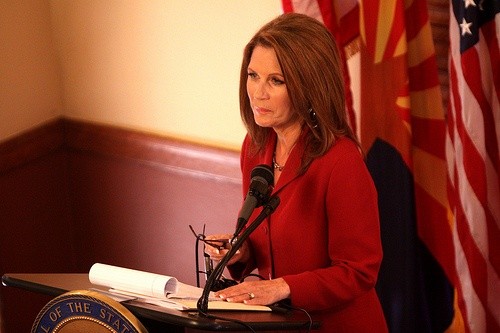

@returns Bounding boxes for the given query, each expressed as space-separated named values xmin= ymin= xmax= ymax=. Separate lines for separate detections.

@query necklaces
xmin=273 ymin=153 xmax=285 ymax=172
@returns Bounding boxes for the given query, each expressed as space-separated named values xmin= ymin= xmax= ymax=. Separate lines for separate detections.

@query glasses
xmin=189 ymin=224 xmax=237 ymax=250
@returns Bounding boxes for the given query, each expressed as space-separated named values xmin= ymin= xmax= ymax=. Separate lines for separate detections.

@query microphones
xmin=234 ymin=164 xmax=274 ymax=237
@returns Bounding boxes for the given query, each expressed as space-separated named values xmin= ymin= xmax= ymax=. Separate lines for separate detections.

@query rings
xmin=248 ymin=292 xmax=256 ymax=300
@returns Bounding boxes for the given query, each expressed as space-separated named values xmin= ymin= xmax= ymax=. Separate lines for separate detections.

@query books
xmin=89 ymin=262 xmax=272 ymax=312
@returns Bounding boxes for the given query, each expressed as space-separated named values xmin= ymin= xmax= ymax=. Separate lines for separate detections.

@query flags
xmin=282 ymin=0 xmax=500 ymax=333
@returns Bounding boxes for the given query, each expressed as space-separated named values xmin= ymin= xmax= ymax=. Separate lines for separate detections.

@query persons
xmin=203 ymin=12 xmax=384 ymax=333
